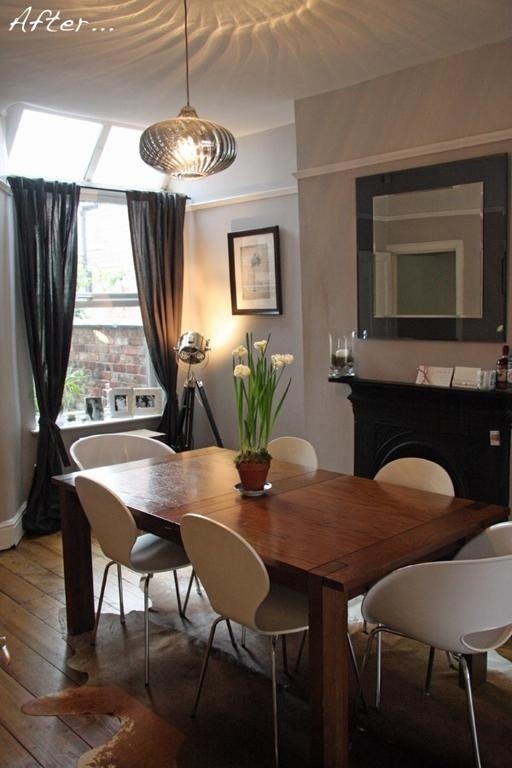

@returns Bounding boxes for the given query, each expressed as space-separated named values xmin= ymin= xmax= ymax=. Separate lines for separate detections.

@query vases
xmin=233 ymin=448 xmax=273 ymax=497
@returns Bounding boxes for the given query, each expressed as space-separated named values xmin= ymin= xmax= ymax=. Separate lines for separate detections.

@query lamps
xmin=138 ymin=2 xmax=237 ymax=181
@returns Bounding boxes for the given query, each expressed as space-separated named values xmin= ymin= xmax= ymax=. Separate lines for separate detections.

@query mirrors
xmin=370 ymin=182 xmax=486 ymax=321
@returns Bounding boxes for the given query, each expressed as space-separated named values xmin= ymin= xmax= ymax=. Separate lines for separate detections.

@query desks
xmin=54 ymin=444 xmax=502 ymax=768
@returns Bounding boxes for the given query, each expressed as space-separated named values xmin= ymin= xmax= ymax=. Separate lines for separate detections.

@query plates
xmin=234 ymin=482 xmax=272 ymax=497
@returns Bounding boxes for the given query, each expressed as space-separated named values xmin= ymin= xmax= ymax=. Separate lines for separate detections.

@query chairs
xmin=179 ymin=512 xmax=358 ymax=768
xmin=454 ymin=520 xmax=512 ymax=560
xmin=74 ymin=475 xmax=238 ymax=687
xmin=373 ymin=456 xmax=454 ymax=499
xmin=69 ymin=434 xmax=200 ymax=613
xmin=358 ymin=555 xmax=511 ymax=768
xmin=265 ymin=435 xmax=317 ymax=469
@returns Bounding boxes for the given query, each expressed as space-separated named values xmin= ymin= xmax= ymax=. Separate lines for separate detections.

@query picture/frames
xmin=226 ymin=225 xmax=284 ymax=317
xmin=83 ymin=396 xmax=104 ymax=422
xmin=131 ymin=388 xmax=162 ymax=415
xmin=107 ymin=387 xmax=133 ymax=417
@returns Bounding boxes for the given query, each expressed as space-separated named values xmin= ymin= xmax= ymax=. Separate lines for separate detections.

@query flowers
xmin=230 ymin=332 xmax=294 ymax=456
